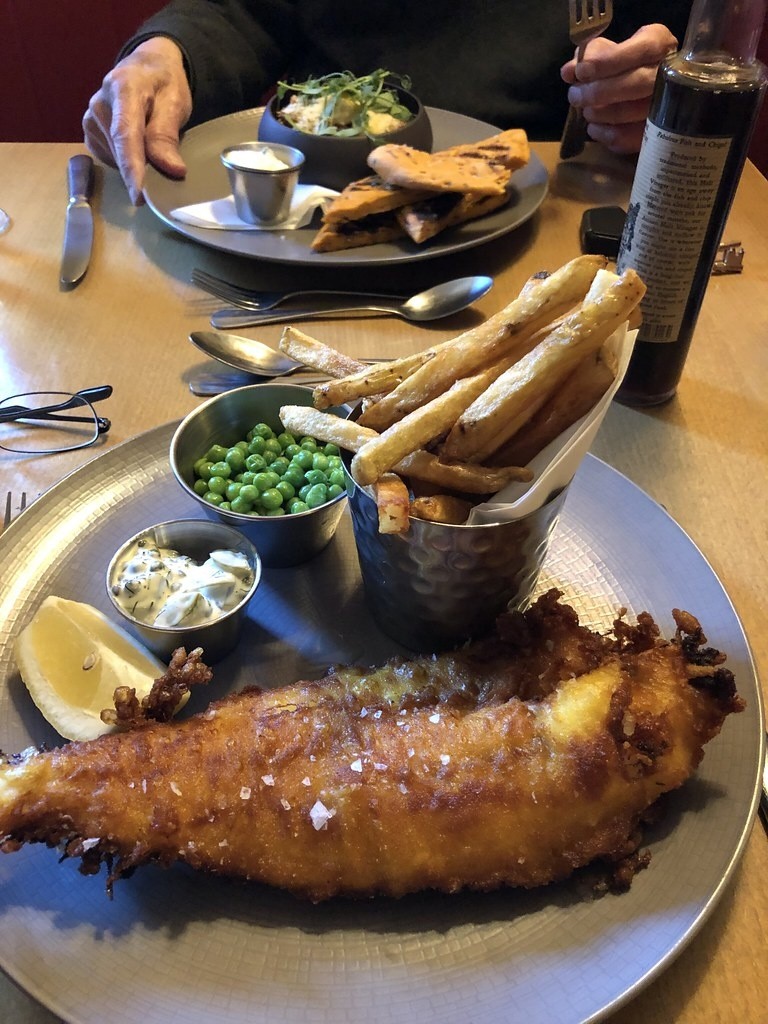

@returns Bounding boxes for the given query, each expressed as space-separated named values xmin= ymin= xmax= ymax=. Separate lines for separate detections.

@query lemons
xmin=13 ymin=594 xmax=194 ymax=746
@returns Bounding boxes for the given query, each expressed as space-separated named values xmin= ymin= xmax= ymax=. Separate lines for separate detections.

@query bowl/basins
xmin=258 ymin=79 xmax=433 ymax=192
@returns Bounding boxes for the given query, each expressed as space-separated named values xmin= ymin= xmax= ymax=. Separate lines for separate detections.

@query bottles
xmin=602 ymin=1 xmax=768 ymax=408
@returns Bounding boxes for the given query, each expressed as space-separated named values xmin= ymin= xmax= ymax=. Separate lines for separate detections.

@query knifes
xmin=59 ymin=154 xmax=94 ymax=284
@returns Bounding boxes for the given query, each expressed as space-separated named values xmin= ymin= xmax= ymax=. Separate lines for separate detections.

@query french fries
xmin=279 ymin=254 xmax=645 ymax=538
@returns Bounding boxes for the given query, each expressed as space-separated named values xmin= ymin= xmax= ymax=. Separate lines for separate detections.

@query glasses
xmin=0 ymin=383 xmax=112 ymax=454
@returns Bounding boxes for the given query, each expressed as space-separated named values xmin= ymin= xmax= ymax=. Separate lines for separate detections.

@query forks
xmin=189 ymin=267 xmax=414 ymax=312
xmin=559 ymin=0 xmax=613 ymax=158
xmin=4 ymin=491 xmax=41 ymax=531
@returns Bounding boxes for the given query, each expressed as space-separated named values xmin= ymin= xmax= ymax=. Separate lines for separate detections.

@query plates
xmin=143 ymin=104 xmax=550 ymax=266
xmin=1 ymin=404 xmax=764 ymax=1023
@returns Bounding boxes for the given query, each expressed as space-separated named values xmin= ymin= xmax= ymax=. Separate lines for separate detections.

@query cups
xmin=220 ymin=140 xmax=305 ymax=226
xmin=106 ymin=517 xmax=263 ymax=667
xmin=339 ymin=402 xmax=576 ymax=652
xmin=170 ymin=385 xmax=358 ymax=565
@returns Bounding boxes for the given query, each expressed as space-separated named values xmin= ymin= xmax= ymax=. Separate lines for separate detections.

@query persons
xmin=81 ymin=0 xmax=694 ymax=207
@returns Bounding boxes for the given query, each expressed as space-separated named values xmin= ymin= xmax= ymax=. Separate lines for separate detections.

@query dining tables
xmin=2 ymin=127 xmax=768 ymax=1024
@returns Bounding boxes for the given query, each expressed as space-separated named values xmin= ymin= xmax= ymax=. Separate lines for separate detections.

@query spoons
xmin=189 ymin=331 xmax=398 ymax=376
xmin=210 ymin=275 xmax=493 ymax=329
